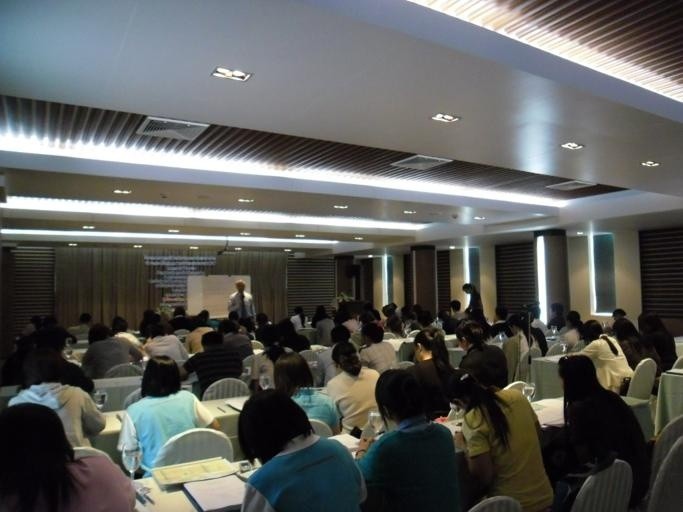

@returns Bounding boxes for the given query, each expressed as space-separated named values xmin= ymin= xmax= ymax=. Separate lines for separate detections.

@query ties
xmin=241 ymin=295 xmax=246 ymax=319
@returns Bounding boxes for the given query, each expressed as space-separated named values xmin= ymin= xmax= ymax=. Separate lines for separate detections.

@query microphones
xmin=239 ymin=287 xmax=244 ymax=298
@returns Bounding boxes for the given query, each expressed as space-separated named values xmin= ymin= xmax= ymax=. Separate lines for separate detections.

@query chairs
xmin=0 ymin=320 xmax=683 ymax=512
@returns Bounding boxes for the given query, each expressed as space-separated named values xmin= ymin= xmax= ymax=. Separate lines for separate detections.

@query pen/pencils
xmin=144 ymin=495 xmax=155 ymax=506
xmin=217 ymin=407 xmax=226 ymax=413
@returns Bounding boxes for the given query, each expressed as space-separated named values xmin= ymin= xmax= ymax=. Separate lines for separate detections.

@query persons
xmin=1 ymin=277 xmax=682 ymax=512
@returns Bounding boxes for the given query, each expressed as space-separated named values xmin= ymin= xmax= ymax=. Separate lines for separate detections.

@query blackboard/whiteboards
xmin=186 ymin=275 xmax=251 ymax=319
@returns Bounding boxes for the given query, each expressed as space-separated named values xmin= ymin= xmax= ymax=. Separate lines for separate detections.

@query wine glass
xmin=92 ymin=390 xmax=107 ymax=412
xmin=64 ymin=347 xmax=73 ymax=361
xmin=122 ymin=440 xmax=143 ymax=492
xmin=367 ymin=409 xmax=384 ymax=437
xmin=258 ymin=375 xmax=270 ymax=390
xmin=551 ymin=326 xmax=557 ymax=338
xmin=498 ymin=331 xmax=504 ymax=343
xmin=523 ymin=381 xmax=536 ymax=403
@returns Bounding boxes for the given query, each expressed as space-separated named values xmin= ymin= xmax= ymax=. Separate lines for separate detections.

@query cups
xmin=560 ymin=342 xmax=567 ymax=353
